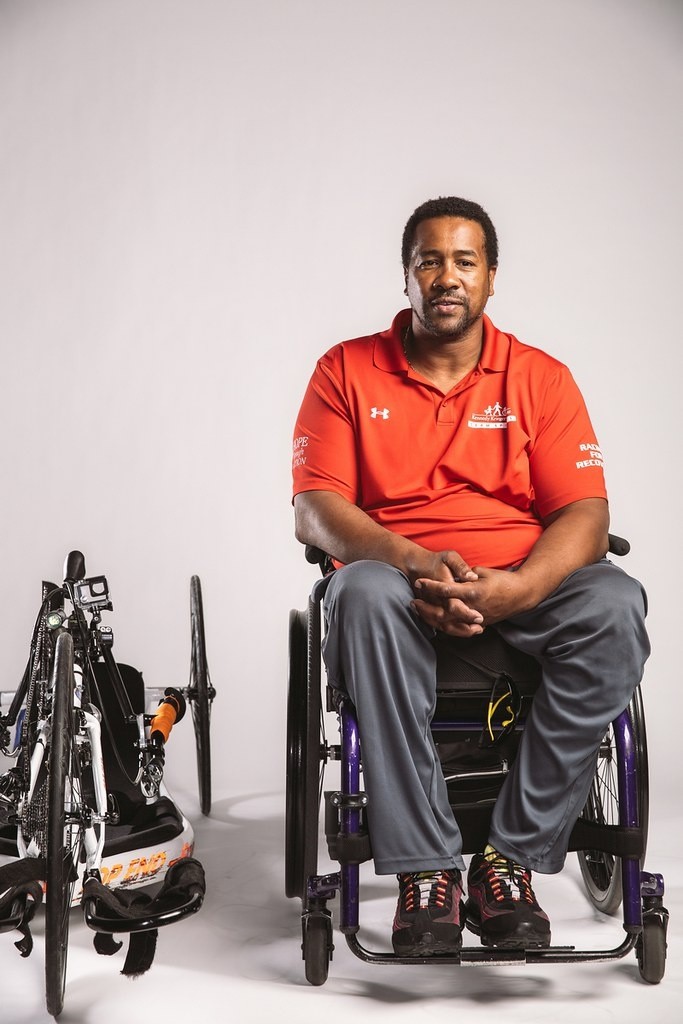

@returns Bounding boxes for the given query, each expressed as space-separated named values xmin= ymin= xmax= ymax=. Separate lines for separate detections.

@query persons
xmin=288 ymin=196 xmax=652 ymax=959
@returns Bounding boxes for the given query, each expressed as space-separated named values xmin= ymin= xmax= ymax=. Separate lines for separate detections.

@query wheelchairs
xmin=0 ymin=550 xmax=217 ymax=1015
xmin=284 ymin=536 xmax=670 ymax=986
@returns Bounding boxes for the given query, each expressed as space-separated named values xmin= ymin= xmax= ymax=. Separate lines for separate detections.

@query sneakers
xmin=392 ymin=868 xmax=462 ymax=953
xmin=462 ymin=849 xmax=552 ymax=949
